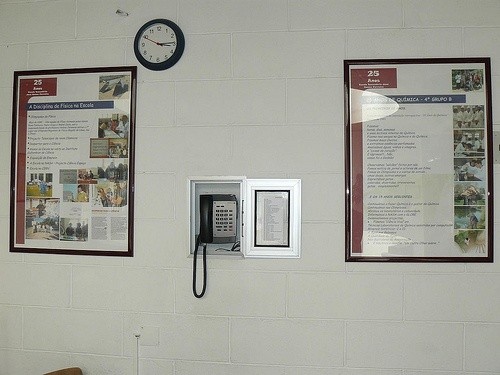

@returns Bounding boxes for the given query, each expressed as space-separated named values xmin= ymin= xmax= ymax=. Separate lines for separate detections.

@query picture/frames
xmin=343 ymin=58 xmax=496 ymax=264
xmin=8 ymin=66 xmax=139 ymax=258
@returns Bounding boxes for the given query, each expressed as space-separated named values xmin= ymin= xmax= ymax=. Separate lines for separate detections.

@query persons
xmin=26 ymin=112 xmax=129 ymax=242
xmin=452 ymin=69 xmax=488 ymax=252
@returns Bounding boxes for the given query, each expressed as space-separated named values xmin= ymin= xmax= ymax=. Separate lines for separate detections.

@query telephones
xmin=199 ymin=194 xmax=238 ymax=244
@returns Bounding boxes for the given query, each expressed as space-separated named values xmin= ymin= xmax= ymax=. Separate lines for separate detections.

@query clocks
xmin=131 ymin=18 xmax=187 ymax=73
